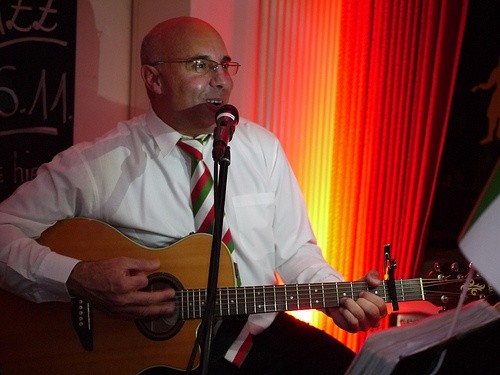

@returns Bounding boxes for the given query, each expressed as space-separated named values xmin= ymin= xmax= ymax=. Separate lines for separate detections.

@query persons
xmin=0 ymin=16 xmax=386 ymax=375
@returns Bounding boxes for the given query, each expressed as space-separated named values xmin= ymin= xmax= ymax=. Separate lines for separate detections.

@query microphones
xmin=211 ymin=104 xmax=239 ymax=162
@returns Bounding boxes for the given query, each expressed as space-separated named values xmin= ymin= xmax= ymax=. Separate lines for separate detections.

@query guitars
xmin=0 ymin=215 xmax=488 ymax=375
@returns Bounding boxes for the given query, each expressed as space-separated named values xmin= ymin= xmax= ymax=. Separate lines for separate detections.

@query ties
xmin=176 ymin=135 xmax=242 ymax=287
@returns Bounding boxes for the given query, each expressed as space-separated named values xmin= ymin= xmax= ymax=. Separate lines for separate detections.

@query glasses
xmin=153 ymin=58 xmax=241 ymax=77
